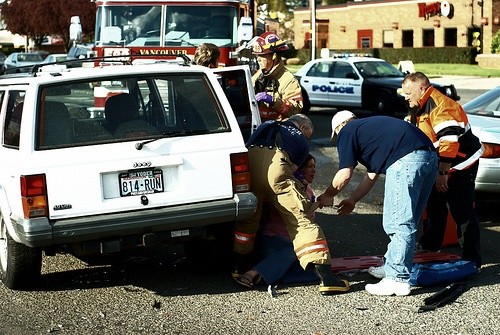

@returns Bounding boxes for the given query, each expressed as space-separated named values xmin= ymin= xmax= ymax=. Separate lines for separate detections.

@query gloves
xmin=255 ymin=92 xmax=272 ymax=103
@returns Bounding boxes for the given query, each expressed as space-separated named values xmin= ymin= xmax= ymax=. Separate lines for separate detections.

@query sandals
xmin=233 ymin=274 xmax=254 ymax=288
xmin=255 ymin=276 xmax=262 ymax=285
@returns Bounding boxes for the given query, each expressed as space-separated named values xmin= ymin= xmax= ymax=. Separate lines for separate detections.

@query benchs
xmin=43 ymin=101 xmax=161 ymax=145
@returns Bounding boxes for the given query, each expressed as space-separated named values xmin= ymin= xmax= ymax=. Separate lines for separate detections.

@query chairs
xmin=101 ymin=93 xmax=139 ymax=134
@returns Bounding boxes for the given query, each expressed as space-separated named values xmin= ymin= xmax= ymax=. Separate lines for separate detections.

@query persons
xmin=232 ymin=114 xmax=350 ymax=295
xmin=400 ymin=71 xmax=485 ymax=270
xmin=251 ymin=32 xmax=304 ymax=124
xmin=193 ymin=42 xmax=230 ymax=99
xmin=317 ymin=110 xmax=440 ymax=297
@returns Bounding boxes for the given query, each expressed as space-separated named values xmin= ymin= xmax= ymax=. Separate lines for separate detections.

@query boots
xmin=313 ymin=264 xmax=350 ymax=295
xmin=231 ymin=251 xmax=252 ymax=277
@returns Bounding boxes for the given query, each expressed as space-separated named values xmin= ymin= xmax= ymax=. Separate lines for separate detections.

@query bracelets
xmin=439 ymin=170 xmax=448 ymax=175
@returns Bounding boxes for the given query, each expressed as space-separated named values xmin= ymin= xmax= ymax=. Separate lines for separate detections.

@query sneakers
xmin=368 ymin=265 xmax=386 ymax=278
xmin=365 ymin=278 xmax=411 ymax=296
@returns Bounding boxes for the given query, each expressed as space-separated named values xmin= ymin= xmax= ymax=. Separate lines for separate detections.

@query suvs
xmin=0 ymin=48 xmax=266 ymax=290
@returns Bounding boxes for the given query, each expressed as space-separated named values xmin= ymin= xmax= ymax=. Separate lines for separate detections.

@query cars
xmin=0 ymin=42 xmax=94 ymax=77
xmin=293 ymin=47 xmax=462 ymax=119
xmin=461 ymin=86 xmax=500 ymax=193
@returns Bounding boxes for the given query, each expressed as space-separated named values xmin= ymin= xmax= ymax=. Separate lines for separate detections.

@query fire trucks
xmin=85 ymin=0 xmax=276 ymax=118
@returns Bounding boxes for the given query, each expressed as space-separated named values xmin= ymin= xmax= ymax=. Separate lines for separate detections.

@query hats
xmin=330 ymin=110 xmax=356 ymax=142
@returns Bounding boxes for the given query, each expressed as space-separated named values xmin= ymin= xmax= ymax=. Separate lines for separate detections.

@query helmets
xmin=252 ymin=32 xmax=288 ymax=55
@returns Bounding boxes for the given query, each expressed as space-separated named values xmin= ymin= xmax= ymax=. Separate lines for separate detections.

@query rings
xmin=442 ymin=185 xmax=444 ymax=188
